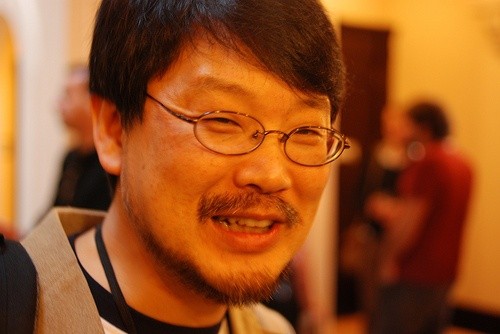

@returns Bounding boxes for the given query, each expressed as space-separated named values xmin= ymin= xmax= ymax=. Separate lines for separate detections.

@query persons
xmin=0 ymin=0 xmax=347 ymax=334
xmin=364 ymin=100 xmax=475 ymax=334
xmin=30 ymin=70 xmax=116 ymax=232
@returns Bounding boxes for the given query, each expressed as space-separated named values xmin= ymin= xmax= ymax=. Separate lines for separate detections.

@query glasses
xmin=144 ymin=91 xmax=352 ymax=168
